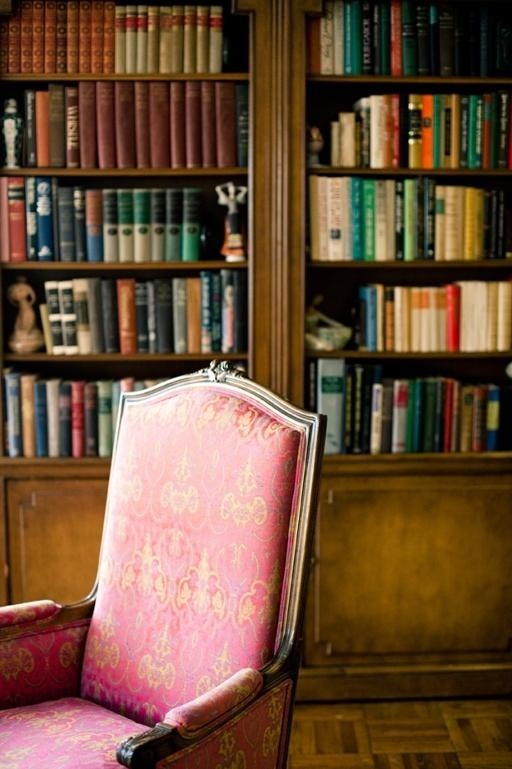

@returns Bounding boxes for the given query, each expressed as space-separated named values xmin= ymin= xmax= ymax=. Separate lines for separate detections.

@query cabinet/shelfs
xmin=0 ymin=1 xmax=512 ymax=457
xmin=0 ymin=458 xmax=512 ymax=698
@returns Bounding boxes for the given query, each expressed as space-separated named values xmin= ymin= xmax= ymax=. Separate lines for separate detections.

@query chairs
xmin=1 ymin=367 xmax=324 ymax=768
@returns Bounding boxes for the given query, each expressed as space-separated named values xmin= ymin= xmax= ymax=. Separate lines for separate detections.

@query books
xmin=352 ymin=279 xmax=509 ymax=353
xmin=0 ymin=174 xmax=210 ymax=263
xmin=3 ymin=78 xmax=247 ymax=172
xmin=325 ymin=80 xmax=511 ymax=166
xmin=38 ymin=272 xmax=249 ymax=362
xmin=1 ymin=0 xmax=250 ymax=74
xmin=309 ymin=2 xmax=510 ymax=75
xmin=305 ymin=175 xmax=509 ymax=263
xmin=2 ymin=373 xmax=188 ymax=458
xmin=304 ymin=358 xmax=512 ymax=455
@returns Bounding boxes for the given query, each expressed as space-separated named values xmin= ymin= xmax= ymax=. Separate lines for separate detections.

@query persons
xmin=305 ymin=292 xmax=351 ymax=352
xmin=214 ymin=180 xmax=246 ymax=262
xmin=3 ymin=274 xmax=42 ymax=356
